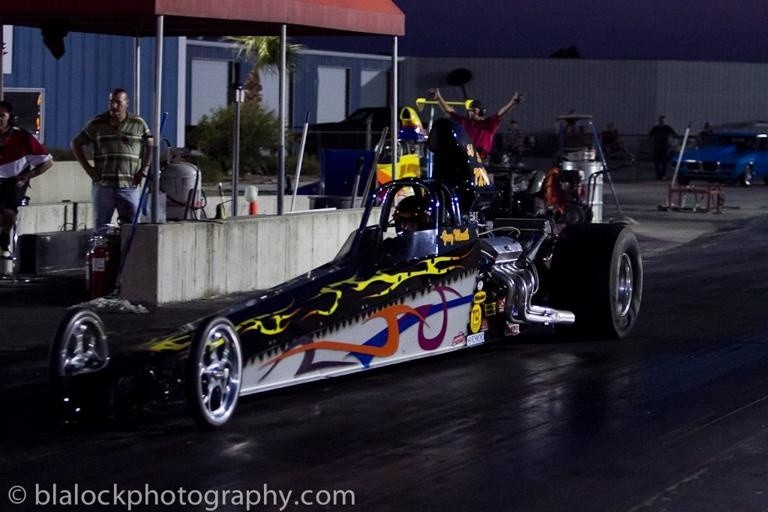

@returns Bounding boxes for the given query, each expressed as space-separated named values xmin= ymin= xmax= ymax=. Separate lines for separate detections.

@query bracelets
xmin=138 ymin=170 xmax=149 ymax=177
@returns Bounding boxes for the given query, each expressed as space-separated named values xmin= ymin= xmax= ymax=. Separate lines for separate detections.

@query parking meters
xmin=229 ymin=82 xmax=247 ymax=220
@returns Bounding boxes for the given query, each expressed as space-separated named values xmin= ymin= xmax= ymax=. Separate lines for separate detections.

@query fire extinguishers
xmin=90 ymin=234 xmax=106 ymax=298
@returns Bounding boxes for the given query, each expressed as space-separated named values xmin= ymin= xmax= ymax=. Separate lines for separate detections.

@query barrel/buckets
xmin=561 ymin=160 xmax=604 ymax=223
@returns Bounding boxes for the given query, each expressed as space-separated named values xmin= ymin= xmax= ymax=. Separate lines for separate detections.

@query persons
xmin=0 ymin=97 xmax=55 ymax=262
xmin=697 ymin=121 xmax=716 ymax=145
xmin=646 ymin=115 xmax=682 ymax=168
xmin=390 ymin=193 xmax=438 ymax=246
xmin=68 ymin=87 xmax=156 ymax=228
xmin=425 ymin=84 xmax=524 ymax=168
xmin=597 ymin=121 xmax=625 ymax=162
xmin=500 ymin=119 xmax=527 ymax=157
xmin=562 ymin=109 xmax=578 ymax=138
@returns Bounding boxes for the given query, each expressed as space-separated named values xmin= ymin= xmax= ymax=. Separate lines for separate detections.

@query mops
xmin=63 ymin=111 xmax=167 ymax=314
xmin=590 ymin=120 xmax=641 ymax=225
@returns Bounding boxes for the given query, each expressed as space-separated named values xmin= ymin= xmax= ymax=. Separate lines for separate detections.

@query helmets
xmin=392 ymin=195 xmax=432 ymax=243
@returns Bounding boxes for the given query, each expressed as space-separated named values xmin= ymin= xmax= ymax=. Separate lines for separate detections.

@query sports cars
xmin=37 ymin=112 xmax=651 ymax=432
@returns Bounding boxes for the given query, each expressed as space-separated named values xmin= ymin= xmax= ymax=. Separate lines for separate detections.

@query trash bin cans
xmin=328 ymin=196 xmax=363 ymax=208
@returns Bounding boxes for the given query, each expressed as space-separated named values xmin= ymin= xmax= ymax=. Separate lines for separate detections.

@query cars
xmin=669 ymin=119 xmax=768 ymax=188
